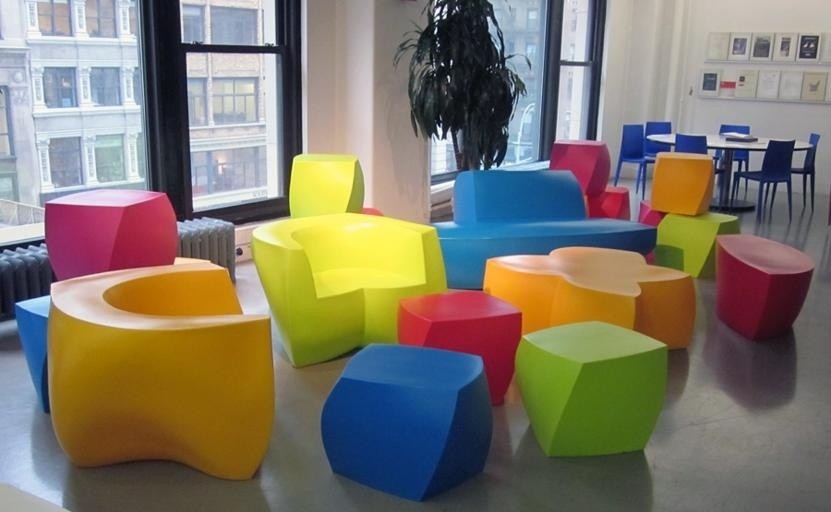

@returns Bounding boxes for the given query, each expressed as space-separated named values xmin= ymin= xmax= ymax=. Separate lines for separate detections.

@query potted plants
xmin=392 ymin=1 xmax=531 ymax=212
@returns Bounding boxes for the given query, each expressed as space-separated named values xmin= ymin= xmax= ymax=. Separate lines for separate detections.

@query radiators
xmin=0 ymin=216 xmax=241 ymax=322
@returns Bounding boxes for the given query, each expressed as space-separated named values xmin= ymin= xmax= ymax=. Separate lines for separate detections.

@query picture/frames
xmin=705 ymin=30 xmax=821 ymax=65
xmin=697 ymin=67 xmax=831 ymax=104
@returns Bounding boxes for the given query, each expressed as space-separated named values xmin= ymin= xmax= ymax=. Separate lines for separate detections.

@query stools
xmin=713 ymin=232 xmax=813 ymax=341
xmin=325 ymin=344 xmax=496 ymax=501
xmin=654 ymin=212 xmax=738 ymax=279
xmin=288 ymin=153 xmax=365 ymax=217
xmin=399 ymin=289 xmax=523 ymax=406
xmin=638 ymin=198 xmax=661 ymax=264
xmin=590 ymin=187 xmax=630 ymax=221
xmin=481 ymin=246 xmax=697 ymax=352
xmin=15 ymin=296 xmax=53 ymax=413
xmin=551 ymin=140 xmax=611 ymax=193
xmin=516 ymin=321 xmax=669 ymax=458
xmin=43 ymin=187 xmax=178 ymax=281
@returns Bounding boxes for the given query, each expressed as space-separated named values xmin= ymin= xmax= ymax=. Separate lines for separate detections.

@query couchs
xmin=429 ymin=169 xmax=656 ymax=291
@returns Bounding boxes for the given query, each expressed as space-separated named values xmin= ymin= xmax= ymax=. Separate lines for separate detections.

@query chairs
xmin=47 ymin=258 xmax=275 ymax=481
xmin=615 ymin=122 xmax=820 ymax=223
xmin=250 ymin=211 xmax=448 ymax=370
